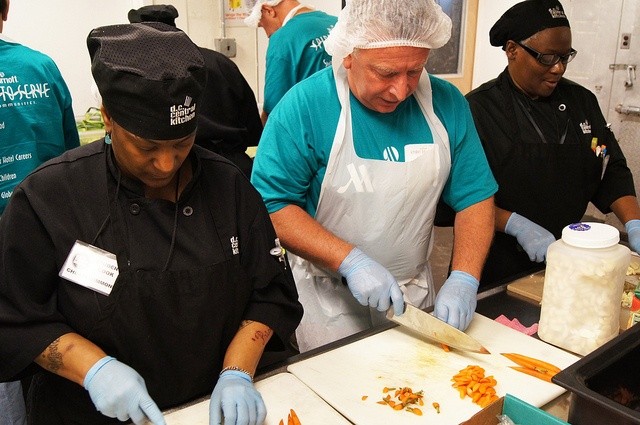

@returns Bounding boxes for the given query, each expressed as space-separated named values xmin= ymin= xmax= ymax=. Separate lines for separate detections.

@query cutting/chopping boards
xmin=507 ymin=254 xmax=639 ymax=334
xmin=287 ymin=310 xmax=581 ymax=425
xmin=145 ymin=372 xmax=353 ymax=425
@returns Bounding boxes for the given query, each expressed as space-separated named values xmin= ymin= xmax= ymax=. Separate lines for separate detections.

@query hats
xmin=244 ymin=0 xmax=283 ymax=29
xmin=489 ymin=0 xmax=570 ymax=51
xmin=128 ymin=5 xmax=178 ymax=28
xmin=323 ymin=0 xmax=452 ymax=58
xmin=87 ymin=22 xmax=206 ymax=140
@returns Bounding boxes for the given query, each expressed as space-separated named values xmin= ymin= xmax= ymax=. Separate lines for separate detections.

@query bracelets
xmin=219 ymin=366 xmax=255 ymax=383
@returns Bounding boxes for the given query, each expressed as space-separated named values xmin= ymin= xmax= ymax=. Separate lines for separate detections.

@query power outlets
xmin=620 ymin=32 xmax=631 ymax=50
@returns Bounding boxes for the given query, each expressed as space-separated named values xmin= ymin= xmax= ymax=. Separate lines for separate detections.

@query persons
xmin=1 ymin=0 xmax=80 ymax=214
xmin=0 ymin=382 xmax=28 ymax=425
xmin=449 ymin=0 xmax=640 ymax=293
xmin=246 ymin=0 xmax=339 ymax=127
xmin=1 ymin=21 xmax=305 ymax=425
xmin=128 ymin=4 xmax=262 ymax=183
xmin=250 ymin=1 xmax=500 ymax=355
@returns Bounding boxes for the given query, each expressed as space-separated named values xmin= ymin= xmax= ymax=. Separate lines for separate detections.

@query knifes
xmin=341 ymin=276 xmax=491 ymax=354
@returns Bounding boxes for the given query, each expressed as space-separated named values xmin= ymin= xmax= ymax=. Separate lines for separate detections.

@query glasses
xmin=516 ymin=42 xmax=577 ymax=66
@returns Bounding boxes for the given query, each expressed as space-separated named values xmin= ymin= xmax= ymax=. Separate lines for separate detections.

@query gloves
xmin=338 ymin=247 xmax=404 ymax=316
xmin=434 ymin=270 xmax=479 ymax=332
xmin=209 ymin=370 xmax=267 ymax=425
xmin=623 ymin=219 xmax=640 ymax=256
xmin=83 ymin=356 xmax=166 ymax=425
xmin=504 ymin=212 xmax=555 ymax=263
